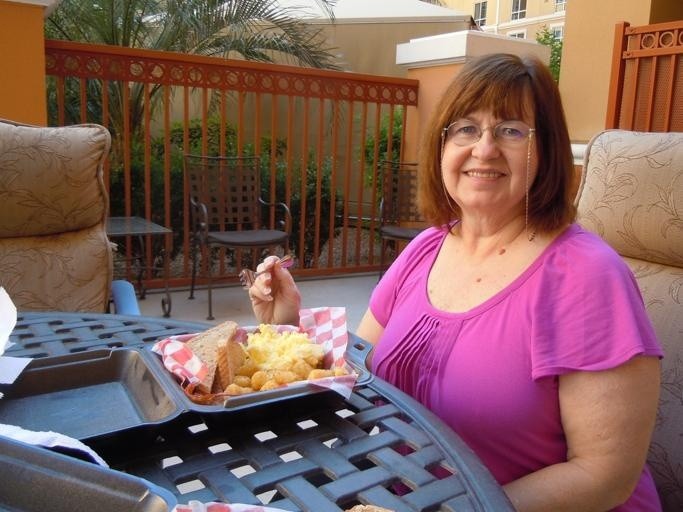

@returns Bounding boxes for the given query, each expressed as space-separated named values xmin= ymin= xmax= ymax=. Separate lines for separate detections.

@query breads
xmin=186 ymin=321 xmax=246 ymax=395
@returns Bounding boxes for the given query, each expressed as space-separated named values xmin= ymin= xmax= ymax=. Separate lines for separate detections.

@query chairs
xmin=573 ymin=129 xmax=683 ymax=510
xmin=377 ymin=157 xmax=433 ymax=283
xmin=0 ymin=117 xmax=115 ymax=311
xmin=181 ymin=153 xmax=294 ymax=320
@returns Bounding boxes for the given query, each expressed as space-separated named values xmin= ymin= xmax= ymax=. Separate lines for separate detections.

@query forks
xmin=238 ymin=253 xmax=295 ymax=291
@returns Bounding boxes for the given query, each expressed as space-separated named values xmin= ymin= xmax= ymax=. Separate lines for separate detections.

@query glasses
xmin=443 ymin=118 xmax=537 ymax=147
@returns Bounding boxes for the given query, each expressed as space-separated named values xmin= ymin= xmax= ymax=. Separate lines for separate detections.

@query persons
xmin=247 ymin=53 xmax=665 ymax=512
